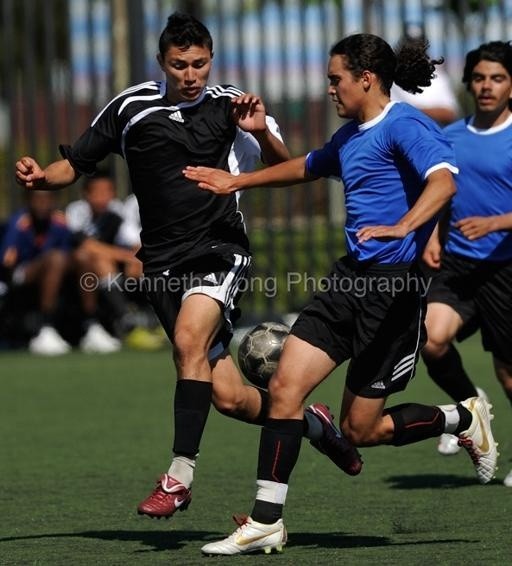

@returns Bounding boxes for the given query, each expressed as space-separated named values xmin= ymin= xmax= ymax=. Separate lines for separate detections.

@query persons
xmin=1 ymin=12 xmax=510 ymax=553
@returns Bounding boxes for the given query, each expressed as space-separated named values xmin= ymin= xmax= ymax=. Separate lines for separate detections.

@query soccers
xmin=238 ymin=321 xmax=291 ymax=388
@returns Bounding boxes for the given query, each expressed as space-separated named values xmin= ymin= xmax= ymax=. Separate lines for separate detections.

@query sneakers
xmin=78 ymin=324 xmax=121 ymax=354
xmin=126 ymin=325 xmax=169 ymax=351
xmin=200 ymin=515 xmax=288 ymax=557
xmin=305 ymin=402 xmax=364 ymax=476
xmin=137 ymin=472 xmax=192 ymax=519
xmin=29 ymin=324 xmax=72 ymax=356
xmin=436 ymin=386 xmax=500 ymax=485
